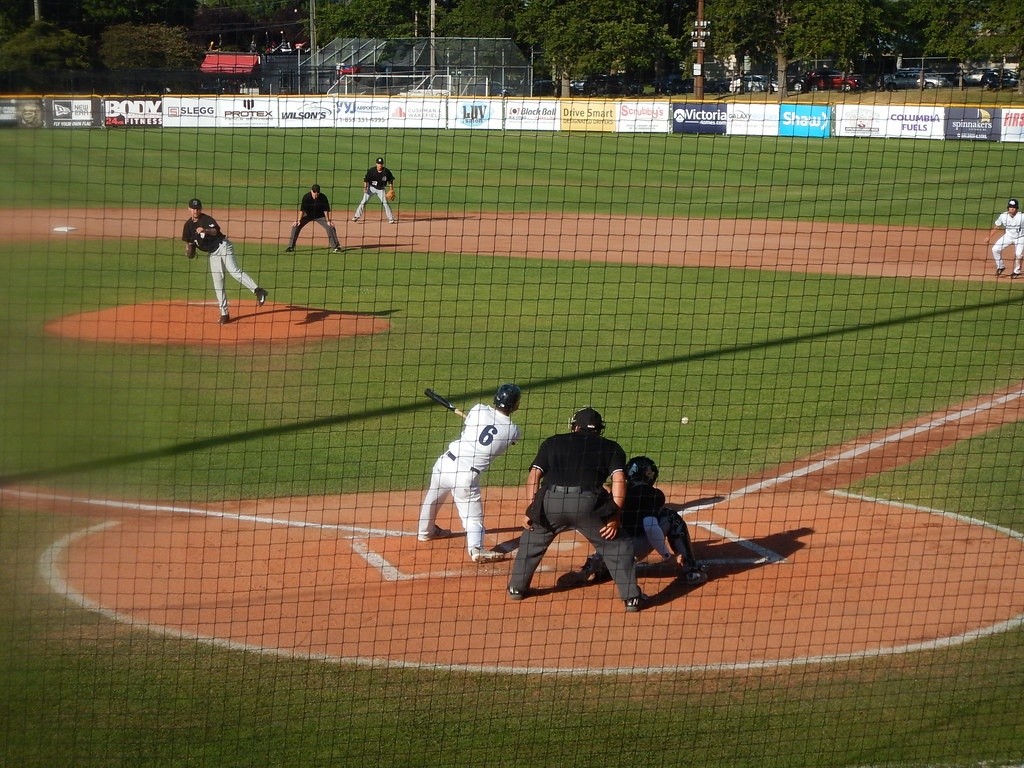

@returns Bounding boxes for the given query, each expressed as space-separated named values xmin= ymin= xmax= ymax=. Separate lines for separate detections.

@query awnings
xmin=199 ymin=54 xmax=259 ymax=76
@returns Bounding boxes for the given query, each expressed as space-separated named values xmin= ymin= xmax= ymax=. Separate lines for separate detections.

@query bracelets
xmin=204 ymin=228 xmax=211 ymax=234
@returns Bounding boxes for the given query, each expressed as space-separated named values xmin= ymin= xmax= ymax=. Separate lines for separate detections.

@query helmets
xmin=624 ymin=456 xmax=658 ymax=486
xmin=494 ymin=384 xmax=520 ymax=409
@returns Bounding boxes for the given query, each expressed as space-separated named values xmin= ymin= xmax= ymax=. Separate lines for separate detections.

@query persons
xmin=984 ymin=199 xmax=1024 ymax=279
xmin=352 ymin=158 xmax=396 ymax=224
xmin=418 ymin=383 xmax=521 ymax=563
xmin=506 ymin=407 xmax=660 ymax=611
xmin=557 ymin=456 xmax=708 ymax=585
xmin=197 ymin=79 xmax=257 ymax=94
xmin=182 ymin=199 xmax=268 ymax=324
xmin=285 ymin=185 xmax=343 ymax=253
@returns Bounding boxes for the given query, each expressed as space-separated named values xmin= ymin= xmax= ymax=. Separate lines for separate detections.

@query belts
xmin=555 ymin=486 xmax=582 ymax=493
xmin=210 ymin=241 xmax=223 ymax=253
xmin=448 ymin=452 xmax=480 ymax=474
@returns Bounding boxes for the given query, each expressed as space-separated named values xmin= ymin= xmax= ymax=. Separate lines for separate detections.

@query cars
xmin=728 ymin=73 xmax=779 ymax=91
xmin=878 ymin=70 xmax=948 ymax=90
xmin=532 ymin=74 xmax=732 ymax=95
xmin=979 ymin=68 xmax=1019 ymax=89
xmin=804 ymin=69 xmax=865 ymax=93
xmin=785 ymin=75 xmax=804 ymax=91
xmin=489 ymin=82 xmax=516 ymax=99
xmin=955 ymin=67 xmax=999 ymax=87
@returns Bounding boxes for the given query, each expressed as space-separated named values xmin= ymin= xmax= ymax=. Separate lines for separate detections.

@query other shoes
xmin=1012 ymin=273 xmax=1020 ymax=279
xmin=352 ymin=217 xmax=359 ymax=221
xmin=997 ymin=268 xmax=1005 ymax=275
xmin=389 ymin=220 xmax=394 ymax=223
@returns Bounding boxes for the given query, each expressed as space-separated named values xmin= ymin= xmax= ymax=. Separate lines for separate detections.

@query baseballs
xmin=682 ymin=417 xmax=689 ymax=425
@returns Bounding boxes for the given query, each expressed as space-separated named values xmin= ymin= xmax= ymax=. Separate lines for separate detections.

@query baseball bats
xmin=424 ymin=388 xmax=516 ymax=445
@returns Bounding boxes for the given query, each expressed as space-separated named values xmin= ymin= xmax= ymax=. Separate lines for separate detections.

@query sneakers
xmin=216 ymin=315 xmax=229 ymax=324
xmin=286 ymin=247 xmax=294 ymax=253
xmin=470 ymin=547 xmax=505 ymax=564
xmin=255 ymin=289 xmax=268 ymax=307
xmin=418 ymin=525 xmax=451 ymax=541
xmin=507 ymin=587 xmax=523 ymax=599
xmin=678 ymin=569 xmax=707 ymax=585
xmin=557 ymin=570 xmax=596 ymax=586
xmin=624 ymin=586 xmax=647 ymax=611
xmin=333 ymin=248 xmax=345 ymax=254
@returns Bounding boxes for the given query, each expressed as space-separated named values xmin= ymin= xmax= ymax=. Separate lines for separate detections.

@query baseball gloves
xmin=187 ymin=242 xmax=196 ymax=258
xmin=386 ymin=190 xmax=396 ymax=202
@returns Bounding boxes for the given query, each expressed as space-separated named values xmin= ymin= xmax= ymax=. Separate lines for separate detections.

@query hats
xmin=573 ymin=408 xmax=604 ymax=428
xmin=189 ymin=199 xmax=202 ymax=209
xmin=1007 ymin=199 xmax=1018 ymax=208
xmin=376 ymin=158 xmax=383 ymax=163
xmin=312 ymin=184 xmax=320 ymax=192
